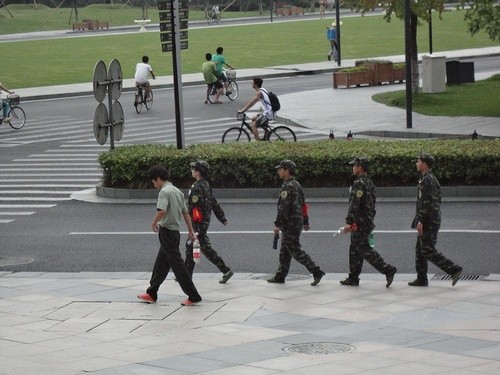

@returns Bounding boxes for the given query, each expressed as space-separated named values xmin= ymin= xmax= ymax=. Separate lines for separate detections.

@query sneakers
xmin=408 ymin=278 xmax=428 ymax=286
xmin=219 ymin=270 xmax=233 ymax=283
xmin=386 ymin=266 xmax=396 ymax=287
xmin=181 ymin=299 xmax=192 ymax=304
xmin=267 ymin=275 xmax=285 ymax=283
xmin=136 ymin=293 xmax=157 ymax=303
xmin=311 ymin=270 xmax=325 ymax=285
xmin=452 ymin=267 xmax=462 ymax=286
xmin=340 ymin=277 xmax=360 ymax=286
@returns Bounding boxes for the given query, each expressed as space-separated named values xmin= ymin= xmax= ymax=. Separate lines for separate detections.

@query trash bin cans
xmin=459 ymin=62 xmax=474 ymax=82
xmin=446 ymin=61 xmax=459 ymax=85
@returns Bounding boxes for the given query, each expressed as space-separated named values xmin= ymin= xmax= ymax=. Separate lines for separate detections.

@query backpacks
xmin=259 ymin=88 xmax=280 ymax=111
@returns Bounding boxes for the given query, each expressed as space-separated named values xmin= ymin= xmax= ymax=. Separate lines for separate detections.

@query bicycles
xmin=0 ymin=92 xmax=26 ymax=129
xmin=208 ymin=10 xmax=222 ymax=25
xmin=207 ymin=68 xmax=239 ymax=105
xmin=221 ymin=112 xmax=298 ymax=143
xmin=134 ymin=84 xmax=154 ymax=115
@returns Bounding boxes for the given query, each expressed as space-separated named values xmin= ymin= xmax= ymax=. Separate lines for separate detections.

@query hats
xmin=189 ymin=160 xmax=210 ymax=172
xmin=332 ymin=20 xmax=342 ymax=26
xmin=275 ymin=160 xmax=296 ymax=172
xmin=348 ymin=156 xmax=370 ymax=166
xmin=412 ymin=155 xmax=431 ymax=162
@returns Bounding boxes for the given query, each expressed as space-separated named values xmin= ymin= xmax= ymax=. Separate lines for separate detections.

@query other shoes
xmin=214 ymin=101 xmax=222 ymax=104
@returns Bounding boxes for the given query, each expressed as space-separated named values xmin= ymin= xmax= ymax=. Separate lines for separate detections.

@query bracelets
xmin=418 ymin=222 xmax=421 ymax=224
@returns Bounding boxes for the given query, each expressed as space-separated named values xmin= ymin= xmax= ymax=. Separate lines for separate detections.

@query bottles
xmin=332 ymin=226 xmax=352 ymax=237
xmin=369 ymin=233 xmax=375 ymax=248
xmin=192 ymin=237 xmax=200 ymax=263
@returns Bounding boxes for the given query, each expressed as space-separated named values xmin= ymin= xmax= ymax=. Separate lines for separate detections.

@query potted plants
xmin=332 ymin=59 xmax=406 ymax=89
xmin=72 ymin=19 xmax=108 ymax=32
xmin=273 ymin=5 xmax=305 ymax=16
xmin=134 ymin=15 xmax=151 ymax=32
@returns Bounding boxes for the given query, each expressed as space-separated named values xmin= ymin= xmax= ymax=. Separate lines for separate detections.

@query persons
xmin=265 ymin=160 xmax=325 ymax=286
xmin=0 ymin=83 xmax=15 ymax=123
xmin=134 ymin=55 xmax=155 ymax=107
xmin=211 ymin=4 xmax=220 ymax=22
xmin=408 ymin=154 xmax=463 ymax=287
xmin=328 ymin=21 xmax=343 ymax=61
xmin=340 ymin=157 xmax=397 ymax=288
xmin=238 ymin=78 xmax=276 ymax=140
xmin=319 ymin=0 xmax=327 ymax=19
xmin=137 ymin=167 xmax=202 ymax=306
xmin=202 ymin=53 xmax=224 ymax=104
xmin=212 ymin=47 xmax=234 ymax=96
xmin=184 ymin=160 xmax=233 ymax=284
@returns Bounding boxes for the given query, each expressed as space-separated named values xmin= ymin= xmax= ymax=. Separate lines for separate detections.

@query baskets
xmin=226 ymin=71 xmax=237 ymax=79
xmin=8 ymin=95 xmax=20 ymax=105
